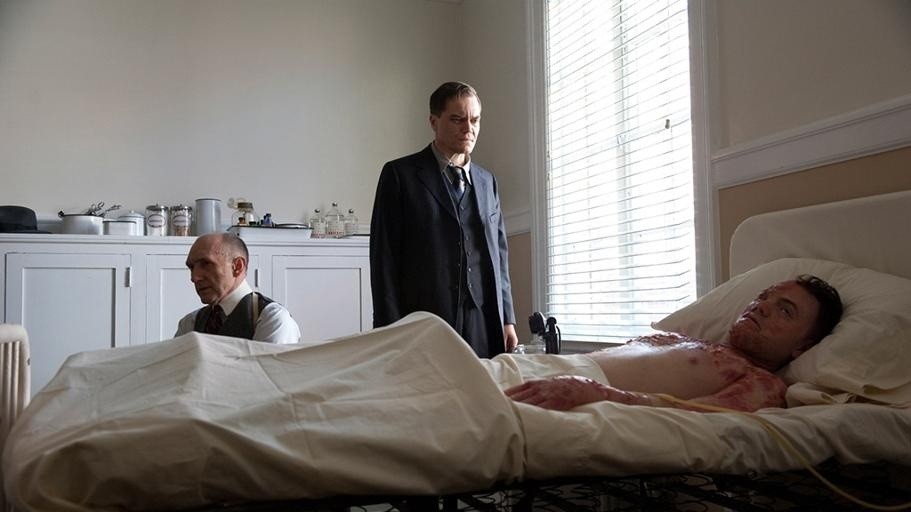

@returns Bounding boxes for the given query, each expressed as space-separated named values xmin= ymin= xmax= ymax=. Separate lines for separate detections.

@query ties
xmin=450 ymin=166 xmax=465 ymax=201
xmin=206 ymin=306 xmax=223 ymax=335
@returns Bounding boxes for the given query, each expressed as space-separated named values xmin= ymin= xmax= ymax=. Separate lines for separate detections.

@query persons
xmin=174 ymin=232 xmax=301 ymax=345
xmin=369 ymin=82 xmax=518 ymax=358
xmin=500 ymin=274 xmax=844 ymax=415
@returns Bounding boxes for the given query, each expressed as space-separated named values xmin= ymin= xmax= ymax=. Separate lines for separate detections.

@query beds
xmin=0 ymin=187 xmax=911 ymax=511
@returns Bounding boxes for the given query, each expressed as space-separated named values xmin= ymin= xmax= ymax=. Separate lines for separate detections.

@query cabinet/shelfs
xmin=267 ymin=235 xmax=374 ymax=345
xmin=3 ymin=237 xmax=144 ymax=405
xmin=143 ymin=236 xmax=270 ymax=344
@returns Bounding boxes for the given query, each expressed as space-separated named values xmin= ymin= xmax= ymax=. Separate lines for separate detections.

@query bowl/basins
xmin=59 ymin=214 xmax=106 ymax=235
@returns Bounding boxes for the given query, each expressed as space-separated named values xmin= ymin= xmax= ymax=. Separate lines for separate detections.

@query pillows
xmin=650 ymin=249 xmax=910 ymax=414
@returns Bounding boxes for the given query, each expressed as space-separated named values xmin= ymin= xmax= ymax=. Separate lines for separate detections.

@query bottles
xmin=311 ymin=202 xmax=359 ymax=239
xmin=120 ymin=197 xmax=280 ymax=235
